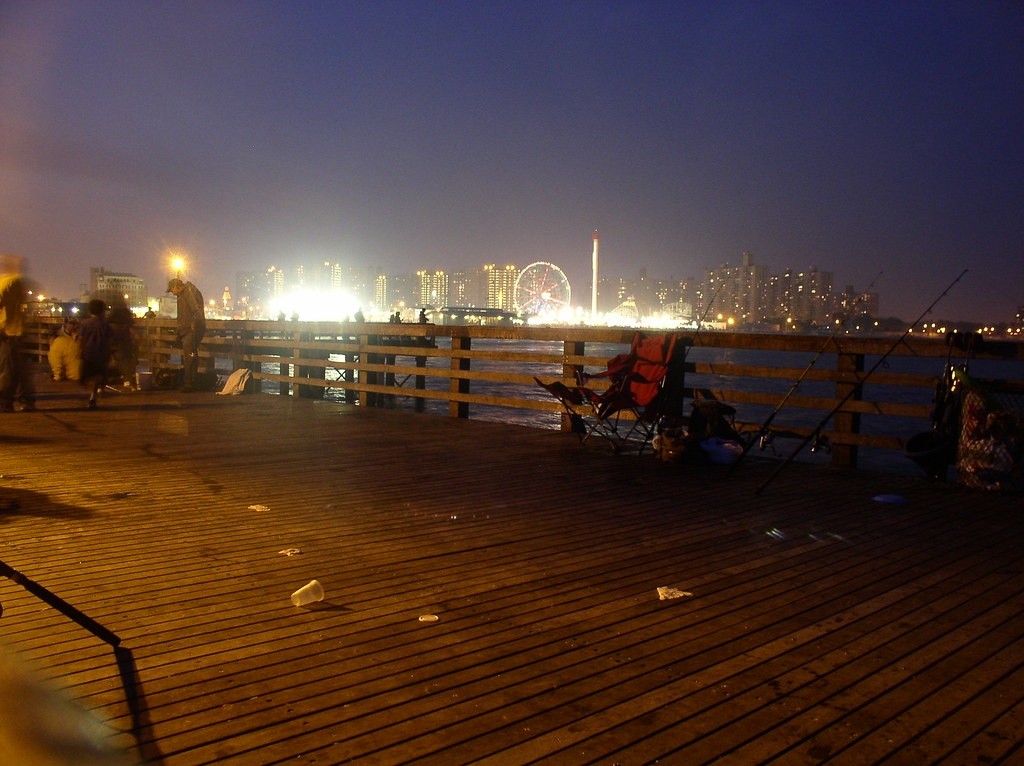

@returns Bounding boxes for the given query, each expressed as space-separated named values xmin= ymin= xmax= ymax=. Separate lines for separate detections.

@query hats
xmin=165 ymin=279 xmax=183 ymax=293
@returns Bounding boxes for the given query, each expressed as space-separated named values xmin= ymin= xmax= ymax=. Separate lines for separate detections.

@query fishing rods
xmin=713 ymin=264 xmax=971 ymax=498
xmin=639 ymin=271 xmax=730 ymax=465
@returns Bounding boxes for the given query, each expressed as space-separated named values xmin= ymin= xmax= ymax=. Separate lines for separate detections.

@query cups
xmin=291 ymin=580 xmax=324 ymax=606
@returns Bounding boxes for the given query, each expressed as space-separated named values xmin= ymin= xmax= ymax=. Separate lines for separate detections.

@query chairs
xmin=534 ymin=331 xmax=685 ymax=473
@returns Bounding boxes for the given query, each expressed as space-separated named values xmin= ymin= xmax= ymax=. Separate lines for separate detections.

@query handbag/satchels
xmin=150 ymin=365 xmax=172 ymax=387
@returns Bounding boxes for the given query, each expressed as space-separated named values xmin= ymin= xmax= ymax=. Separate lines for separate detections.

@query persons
xmin=0 ymin=258 xmax=37 ymax=413
xmin=390 ymin=312 xmax=401 ymax=323
xmin=51 ymin=317 xmax=79 ymax=382
xmin=168 ymin=278 xmax=206 ymax=392
xmin=904 ymin=375 xmax=962 ymax=482
xmin=354 ymin=308 xmax=365 ymax=322
xmin=143 ymin=307 xmax=156 ymax=319
xmin=80 ymin=288 xmax=139 ymax=410
xmin=419 ymin=308 xmax=429 ymax=323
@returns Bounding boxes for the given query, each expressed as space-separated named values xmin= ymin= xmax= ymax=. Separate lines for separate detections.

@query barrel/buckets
xmin=136 ymin=372 xmax=152 ymax=390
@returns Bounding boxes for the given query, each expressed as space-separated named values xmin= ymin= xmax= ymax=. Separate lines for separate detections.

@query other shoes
xmin=97 ymin=388 xmax=106 ymax=398
xmin=87 ymin=394 xmax=96 ymax=411
xmin=179 ymin=387 xmax=198 ymax=392
